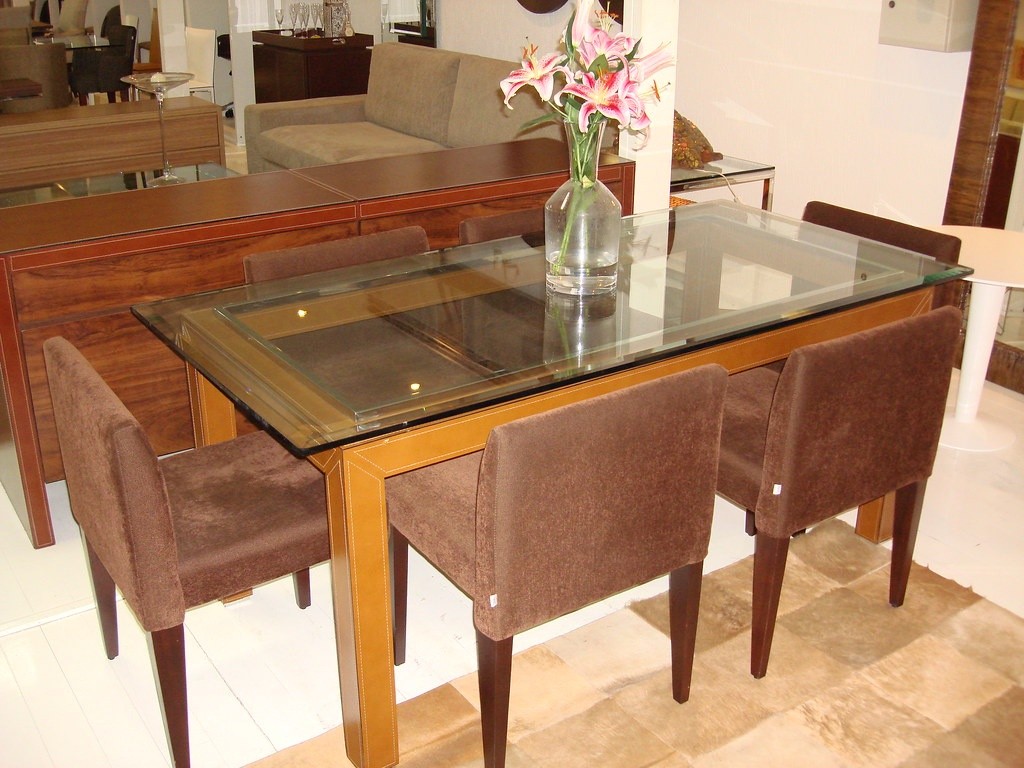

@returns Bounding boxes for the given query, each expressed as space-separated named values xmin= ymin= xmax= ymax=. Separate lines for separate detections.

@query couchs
xmin=245 ymin=42 xmax=566 ymax=174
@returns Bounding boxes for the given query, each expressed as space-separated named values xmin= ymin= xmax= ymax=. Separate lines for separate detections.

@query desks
xmin=32 ymin=21 xmax=53 ymax=29
xmin=671 ymin=155 xmax=775 ymax=211
xmin=131 ymin=199 xmax=974 ymax=768
xmin=0 ymin=96 xmax=226 ymax=189
xmin=33 ymin=35 xmax=125 ymax=49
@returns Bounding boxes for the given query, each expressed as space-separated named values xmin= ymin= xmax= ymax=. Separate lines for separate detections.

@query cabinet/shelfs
xmin=0 ymin=137 xmax=636 ymax=551
xmin=252 ymin=28 xmax=374 ymax=104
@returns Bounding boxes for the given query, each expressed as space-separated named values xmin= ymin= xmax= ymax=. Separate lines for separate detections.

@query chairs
xmin=244 ymin=226 xmax=429 ymax=285
xmin=0 ymin=0 xmax=162 ymax=113
xmin=42 ymin=336 xmax=330 ymax=768
xmin=718 ymin=305 xmax=962 ymax=678
xmin=385 ymin=362 xmax=729 ymax=768
xmin=791 ymin=201 xmax=962 ymax=536
xmin=459 ymin=205 xmax=546 ymax=246
xmin=185 ymin=27 xmax=217 ymax=103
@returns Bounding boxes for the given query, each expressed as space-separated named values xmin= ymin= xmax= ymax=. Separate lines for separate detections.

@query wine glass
xmin=289 ymin=2 xmax=327 ymax=37
xmin=121 ymin=72 xmax=195 ymax=187
xmin=275 ymin=9 xmax=284 ymax=35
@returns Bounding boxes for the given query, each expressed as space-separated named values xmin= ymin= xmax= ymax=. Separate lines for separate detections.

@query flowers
xmin=499 ymin=0 xmax=676 ymax=275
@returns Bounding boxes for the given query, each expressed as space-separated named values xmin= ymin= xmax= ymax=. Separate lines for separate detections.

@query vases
xmin=545 ymin=117 xmax=622 ymax=294
xmin=543 ymin=292 xmax=616 ymax=379
